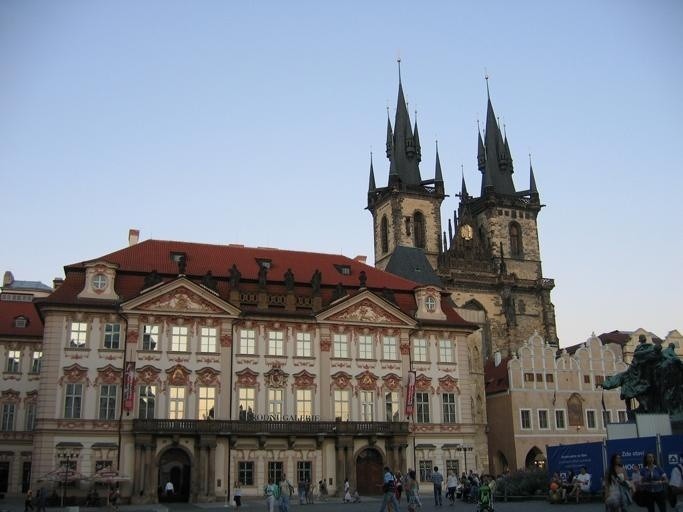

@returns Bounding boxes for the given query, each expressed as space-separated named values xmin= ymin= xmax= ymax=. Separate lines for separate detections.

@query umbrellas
xmin=38 ymin=463 xmax=90 ymax=506
xmin=86 ymin=466 xmax=130 ymax=504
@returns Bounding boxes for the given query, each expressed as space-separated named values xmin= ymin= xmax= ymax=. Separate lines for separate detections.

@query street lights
xmin=63 ymin=448 xmax=71 ymax=508
xmin=407 ymin=412 xmax=416 ymax=479
xmin=456 ymin=444 xmax=474 ymax=475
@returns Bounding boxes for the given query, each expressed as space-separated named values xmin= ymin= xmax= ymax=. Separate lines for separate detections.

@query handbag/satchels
xmin=631 ymin=490 xmax=649 ymax=507
xmin=289 ymin=485 xmax=294 ymax=496
xmin=624 ymin=490 xmax=633 ymax=505
xmin=388 ymin=480 xmax=395 ymax=493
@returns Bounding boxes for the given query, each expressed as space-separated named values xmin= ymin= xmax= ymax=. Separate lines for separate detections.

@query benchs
xmin=553 ymin=488 xmax=606 ymax=502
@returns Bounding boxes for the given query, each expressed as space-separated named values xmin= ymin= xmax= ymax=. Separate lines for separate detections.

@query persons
xmin=233 ymin=482 xmax=241 ymax=512
xmin=24 ymin=482 xmax=121 ymax=512
xmin=165 ymin=480 xmax=175 ymax=503
xmin=632 ymin=334 xmax=681 ymax=368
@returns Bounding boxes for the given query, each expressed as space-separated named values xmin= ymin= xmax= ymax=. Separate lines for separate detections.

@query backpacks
xmin=667 ymin=465 xmax=683 ymax=508
